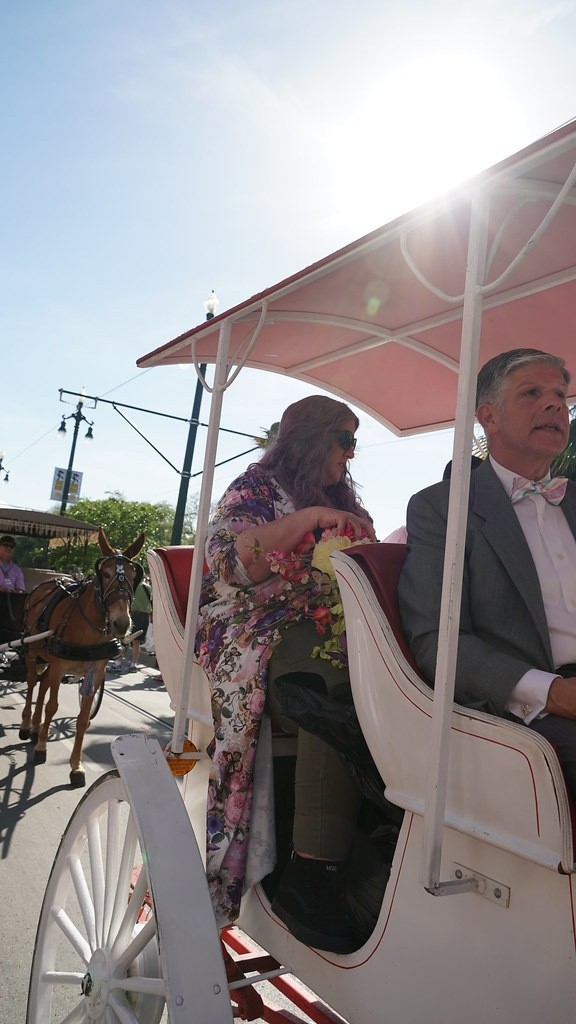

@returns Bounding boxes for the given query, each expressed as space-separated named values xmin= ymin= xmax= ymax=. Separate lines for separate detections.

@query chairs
xmin=147 ymin=545 xmax=215 ymax=731
xmin=329 ymin=542 xmax=575 ymax=877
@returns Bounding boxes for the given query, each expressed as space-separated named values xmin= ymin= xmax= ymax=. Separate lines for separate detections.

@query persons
xmin=0 ymin=534 xmax=26 ymax=592
xmin=400 ymin=346 xmax=576 ymax=791
xmin=382 ymin=454 xmax=483 ymax=543
xmin=191 ymin=395 xmax=378 ymax=941
xmin=105 ymin=581 xmax=152 ymax=672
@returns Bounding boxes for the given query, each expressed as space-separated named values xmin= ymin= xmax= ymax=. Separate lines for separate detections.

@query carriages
xmin=29 ymin=123 xmax=572 ymax=1020
xmin=0 ymin=507 xmax=146 ymax=788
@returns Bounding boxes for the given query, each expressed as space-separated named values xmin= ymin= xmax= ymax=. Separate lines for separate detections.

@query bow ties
xmin=511 ymin=476 xmax=567 ymax=508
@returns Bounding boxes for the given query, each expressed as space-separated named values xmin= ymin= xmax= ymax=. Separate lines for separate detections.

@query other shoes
xmin=271 ymin=852 xmax=363 ymax=952
xmin=109 ymin=664 xmax=137 ymax=673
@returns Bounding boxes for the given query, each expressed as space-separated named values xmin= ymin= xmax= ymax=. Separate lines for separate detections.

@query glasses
xmin=334 ymin=429 xmax=356 ymax=452
xmin=1 ymin=543 xmax=15 ymax=549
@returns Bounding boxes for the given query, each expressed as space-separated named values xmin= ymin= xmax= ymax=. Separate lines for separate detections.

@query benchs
xmin=19 ymin=568 xmax=56 ymax=594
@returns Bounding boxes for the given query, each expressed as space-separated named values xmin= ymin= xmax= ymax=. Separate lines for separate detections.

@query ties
xmin=1 ymin=566 xmax=12 ymax=579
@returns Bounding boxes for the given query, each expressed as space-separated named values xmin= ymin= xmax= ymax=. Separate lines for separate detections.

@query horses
xmin=19 ymin=525 xmax=146 ymax=787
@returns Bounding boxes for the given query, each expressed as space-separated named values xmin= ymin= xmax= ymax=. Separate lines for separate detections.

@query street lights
xmin=54 ymin=287 xmax=272 ymax=545
xmin=56 ymin=386 xmax=94 ymax=516
